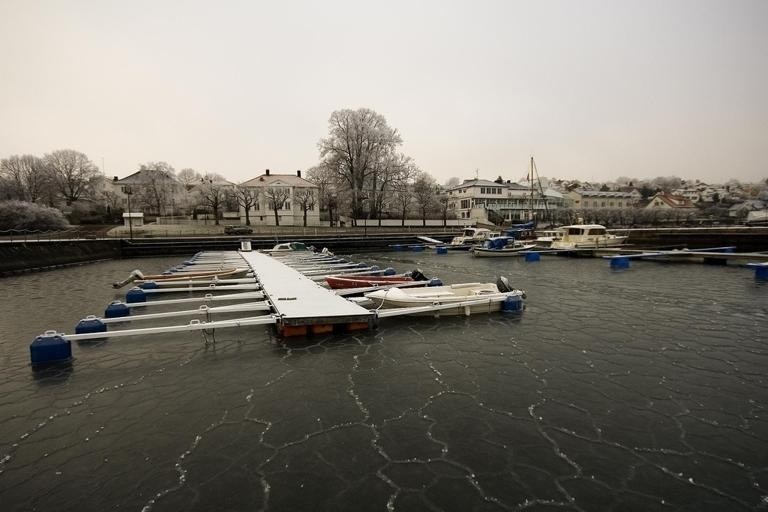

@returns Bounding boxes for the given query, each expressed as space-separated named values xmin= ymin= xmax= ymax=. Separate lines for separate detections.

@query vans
xmin=224 ymin=223 xmax=254 ymax=235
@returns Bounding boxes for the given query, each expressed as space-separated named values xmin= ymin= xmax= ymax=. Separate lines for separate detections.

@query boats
xmin=448 ymin=155 xmax=631 ymax=262
xmin=110 ymin=269 xmax=251 ymax=290
xmin=324 ymin=268 xmax=527 ymax=318
xmin=260 ymin=241 xmax=316 ymax=255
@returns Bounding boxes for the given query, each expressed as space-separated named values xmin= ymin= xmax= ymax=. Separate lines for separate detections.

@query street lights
xmin=119 ymin=184 xmax=133 ymax=240
